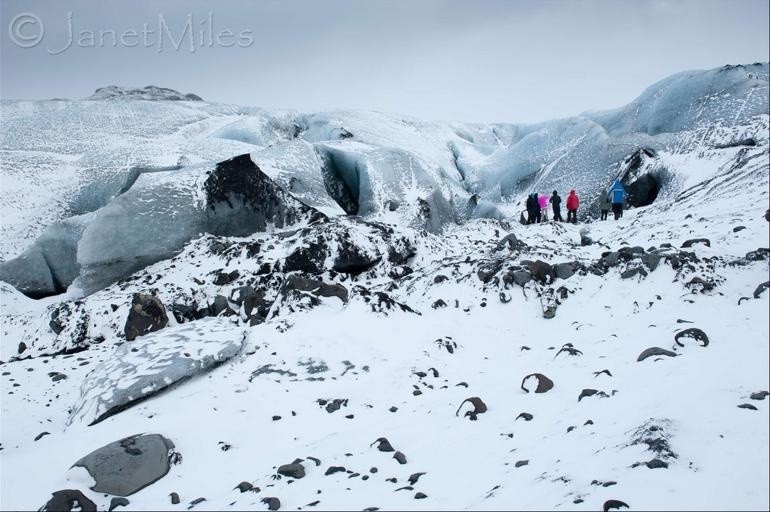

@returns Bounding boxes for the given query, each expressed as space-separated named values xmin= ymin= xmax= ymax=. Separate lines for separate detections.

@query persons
xmin=549 ymin=191 xmax=562 ymax=221
xmin=526 ymin=192 xmax=548 ymax=224
xmin=608 ymin=180 xmax=629 ymax=220
xmin=599 ymin=190 xmax=610 ymax=220
xmin=566 ymin=190 xmax=579 ymax=223
